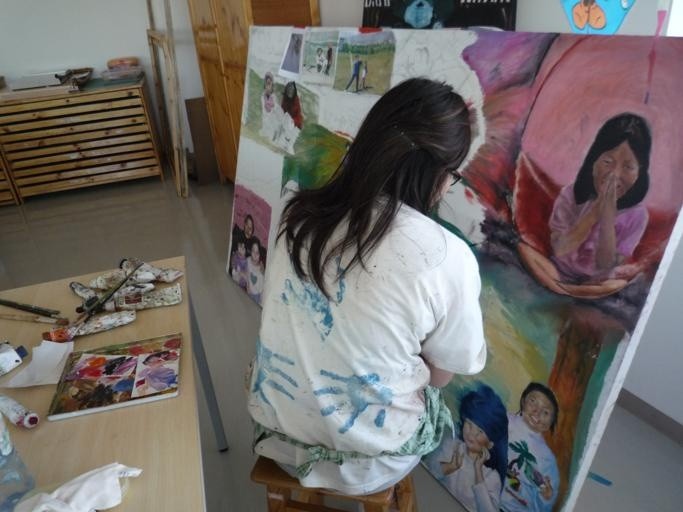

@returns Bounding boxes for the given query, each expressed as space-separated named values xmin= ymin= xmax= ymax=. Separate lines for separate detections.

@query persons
xmin=499 ymin=380 xmax=563 ymax=511
xmin=434 ymin=386 xmax=513 ymax=511
xmin=225 ymin=212 xmax=264 ymax=307
xmin=515 ymin=110 xmax=653 ymax=304
xmin=246 ymin=73 xmax=490 ymax=499
xmin=253 ymin=46 xmax=370 ymax=154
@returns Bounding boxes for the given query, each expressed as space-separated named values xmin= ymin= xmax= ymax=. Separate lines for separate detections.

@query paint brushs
xmin=0 ymin=262 xmax=145 ymax=342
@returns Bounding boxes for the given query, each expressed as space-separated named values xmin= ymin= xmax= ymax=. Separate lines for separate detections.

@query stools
xmin=250 ymin=455 xmax=417 ymax=511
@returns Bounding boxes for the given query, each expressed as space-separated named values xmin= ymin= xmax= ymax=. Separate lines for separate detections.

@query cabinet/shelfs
xmin=187 ymin=0 xmax=321 ymax=185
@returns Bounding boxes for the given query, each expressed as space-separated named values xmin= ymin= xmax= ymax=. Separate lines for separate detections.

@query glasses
xmin=448 ymin=169 xmax=462 ymax=186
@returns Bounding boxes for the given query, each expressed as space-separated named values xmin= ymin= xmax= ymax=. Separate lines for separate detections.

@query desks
xmin=0 ymin=72 xmax=165 ymax=204
xmin=0 ymin=255 xmax=228 ymax=512
xmin=0 ymin=154 xmax=20 ymax=206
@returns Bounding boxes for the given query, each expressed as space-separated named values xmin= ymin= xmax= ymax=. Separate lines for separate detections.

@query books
xmin=43 ymin=330 xmax=183 ymax=423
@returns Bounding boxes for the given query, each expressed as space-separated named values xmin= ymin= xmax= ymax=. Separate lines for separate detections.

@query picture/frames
xmin=225 ymin=25 xmax=683 ymax=512
